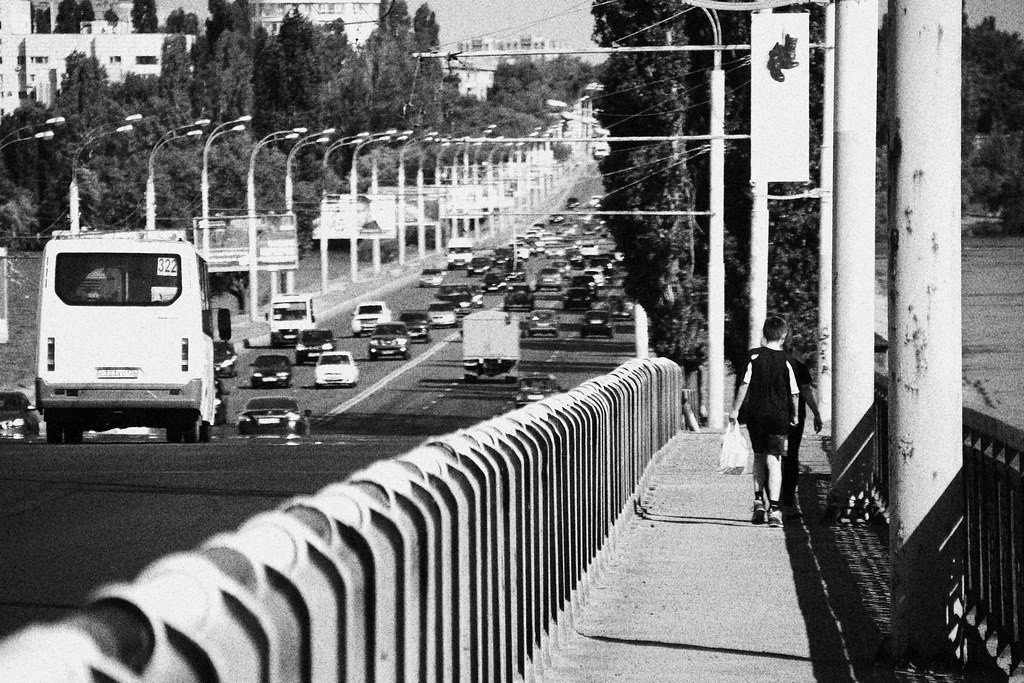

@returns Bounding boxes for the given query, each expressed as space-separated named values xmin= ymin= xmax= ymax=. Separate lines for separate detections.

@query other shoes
xmin=766 ymin=507 xmax=784 ymax=527
xmin=750 ymin=500 xmax=765 ymax=524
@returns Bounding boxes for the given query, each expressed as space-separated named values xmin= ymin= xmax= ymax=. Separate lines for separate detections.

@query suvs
xmin=350 ymin=301 xmax=391 ymax=339
xmin=369 ymin=323 xmax=411 ymax=361
xmin=398 ymin=308 xmax=434 ymax=348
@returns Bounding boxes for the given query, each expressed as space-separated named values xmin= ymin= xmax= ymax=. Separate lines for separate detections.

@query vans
xmin=33 ymin=228 xmax=233 ymax=444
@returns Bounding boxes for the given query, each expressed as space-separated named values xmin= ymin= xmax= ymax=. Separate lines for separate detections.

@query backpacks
xmin=738 ymin=351 xmax=796 ymax=436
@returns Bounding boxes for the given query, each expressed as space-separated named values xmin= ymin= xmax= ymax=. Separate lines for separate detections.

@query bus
xmin=315 ymin=349 xmax=360 ymax=389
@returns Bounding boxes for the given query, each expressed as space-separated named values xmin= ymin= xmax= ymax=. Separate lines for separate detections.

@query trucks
xmin=264 ymin=293 xmax=318 ymax=349
xmin=458 ymin=309 xmax=522 ymax=388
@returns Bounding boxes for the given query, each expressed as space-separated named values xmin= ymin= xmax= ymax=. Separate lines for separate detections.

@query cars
xmin=590 ymin=141 xmax=611 ymax=161
xmin=211 ymin=339 xmax=240 ymax=378
xmin=247 ymin=354 xmax=294 ymax=390
xmin=214 ymin=377 xmax=231 ymax=428
xmin=1 ymin=391 xmax=41 ymax=444
xmin=237 ymin=397 xmax=315 ymax=438
xmin=511 ymin=371 xmax=560 ymax=408
xmin=295 ymin=326 xmax=339 ymax=366
xmin=420 ymin=195 xmax=635 ymax=342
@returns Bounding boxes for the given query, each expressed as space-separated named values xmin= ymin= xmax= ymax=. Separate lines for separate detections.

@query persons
xmin=730 ymin=316 xmax=824 ymax=527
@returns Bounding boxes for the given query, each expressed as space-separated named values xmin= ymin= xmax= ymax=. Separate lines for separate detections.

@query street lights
xmin=348 ymin=129 xmax=399 ymax=286
xmin=320 ymin=131 xmax=370 ymax=294
xmin=284 ymin=127 xmax=339 ymax=292
xmin=248 ymin=126 xmax=307 ymax=323
xmin=397 ymin=132 xmax=438 ymax=268
xmin=201 ymin=113 xmax=251 ymax=261
xmin=146 ymin=118 xmax=212 ymax=231
xmin=372 ymin=132 xmax=409 ymax=276
xmin=69 ymin=113 xmax=144 ymax=232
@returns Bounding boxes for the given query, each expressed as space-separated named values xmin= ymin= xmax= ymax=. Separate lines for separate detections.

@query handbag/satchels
xmin=716 ymin=420 xmax=750 ymax=476
